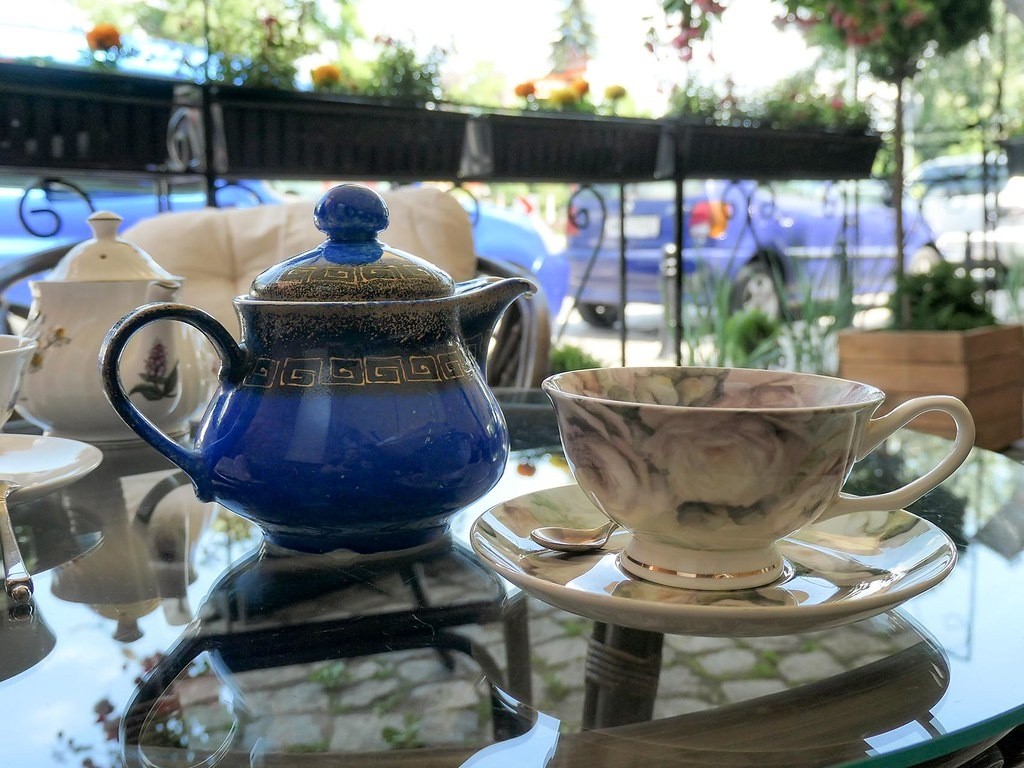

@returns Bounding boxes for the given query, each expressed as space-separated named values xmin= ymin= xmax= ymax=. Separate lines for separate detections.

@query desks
xmin=0 ymin=429 xmax=1024 ymax=768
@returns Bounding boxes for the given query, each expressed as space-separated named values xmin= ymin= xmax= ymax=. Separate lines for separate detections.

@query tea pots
xmin=7 ymin=209 xmax=208 ymax=446
xmin=24 ymin=437 xmax=220 ymax=646
xmin=98 ymin=183 xmax=538 ymax=559
xmin=120 ymin=530 xmax=537 ymax=768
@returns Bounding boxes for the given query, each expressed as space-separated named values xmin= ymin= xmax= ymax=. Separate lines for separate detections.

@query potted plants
xmin=836 ymin=249 xmax=1024 ymax=453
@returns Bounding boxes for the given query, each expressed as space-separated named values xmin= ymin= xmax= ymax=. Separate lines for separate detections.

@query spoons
xmin=0 ymin=479 xmax=34 ymax=603
xmin=534 ymin=515 xmax=619 ymax=553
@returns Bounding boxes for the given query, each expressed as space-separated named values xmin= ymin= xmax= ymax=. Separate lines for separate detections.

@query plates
xmin=470 ymin=487 xmax=958 ymax=636
xmin=470 ymin=589 xmax=951 ymax=752
xmin=0 ymin=500 xmax=107 ymax=584
xmin=0 ymin=434 xmax=104 ymax=511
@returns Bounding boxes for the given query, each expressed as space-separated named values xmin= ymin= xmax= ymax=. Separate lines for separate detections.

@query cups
xmin=540 ymin=367 xmax=977 ymax=591
xmin=1 ymin=333 xmax=39 ymax=433
xmin=0 ymin=579 xmax=58 ymax=684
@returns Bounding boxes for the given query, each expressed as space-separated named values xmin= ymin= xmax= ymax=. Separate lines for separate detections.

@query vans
xmin=908 ymin=155 xmax=1008 ymax=197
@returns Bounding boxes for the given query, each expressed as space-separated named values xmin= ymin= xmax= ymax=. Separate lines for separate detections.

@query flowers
xmin=70 ymin=0 xmax=925 ymax=127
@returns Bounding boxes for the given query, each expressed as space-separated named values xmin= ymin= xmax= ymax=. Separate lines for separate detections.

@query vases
xmin=0 ymin=57 xmax=204 ymax=176
xmin=210 ymin=89 xmax=470 ymax=179
xmin=462 ymin=106 xmax=661 ymax=175
xmin=661 ymin=118 xmax=884 ymax=180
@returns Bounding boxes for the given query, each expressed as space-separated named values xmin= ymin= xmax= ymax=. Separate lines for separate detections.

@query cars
xmin=1 ymin=170 xmax=573 ymax=360
xmin=561 ymin=176 xmax=944 ymax=339
